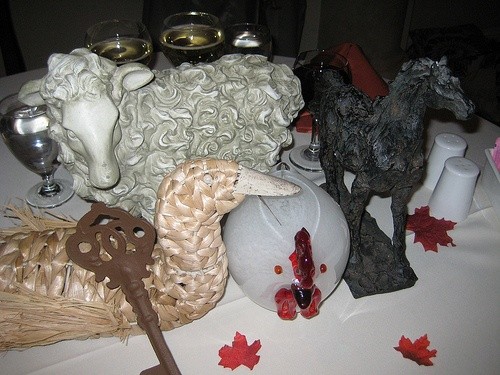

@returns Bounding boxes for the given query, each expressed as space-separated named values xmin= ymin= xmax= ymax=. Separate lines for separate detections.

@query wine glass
xmin=289 ymin=49 xmax=351 ymax=171
xmin=0 ymin=92 xmax=76 ymax=209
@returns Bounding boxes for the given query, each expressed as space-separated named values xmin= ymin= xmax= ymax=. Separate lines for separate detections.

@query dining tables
xmin=0 ymin=48 xmax=500 ymax=375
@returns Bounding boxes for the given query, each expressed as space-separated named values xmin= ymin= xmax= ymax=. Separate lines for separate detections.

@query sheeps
xmin=18 ymin=48 xmax=304 ymax=226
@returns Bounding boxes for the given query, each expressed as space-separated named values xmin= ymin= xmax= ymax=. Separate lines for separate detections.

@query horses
xmin=319 ymin=55 xmax=475 ymax=278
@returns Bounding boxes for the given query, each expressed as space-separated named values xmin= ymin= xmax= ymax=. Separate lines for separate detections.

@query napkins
xmin=295 ymin=43 xmax=389 ymax=133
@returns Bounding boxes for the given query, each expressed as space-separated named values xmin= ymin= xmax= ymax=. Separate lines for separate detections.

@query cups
xmin=85 ymin=17 xmax=155 ymax=70
xmin=428 ymin=156 xmax=480 ymax=223
xmin=424 ymin=133 xmax=467 ymax=190
xmin=222 ymin=7 xmax=274 ymax=63
xmin=158 ymin=11 xmax=227 ymax=68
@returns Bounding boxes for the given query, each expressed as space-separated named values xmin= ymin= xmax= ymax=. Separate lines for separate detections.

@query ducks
xmin=0 ymin=156 xmax=302 ymax=355
